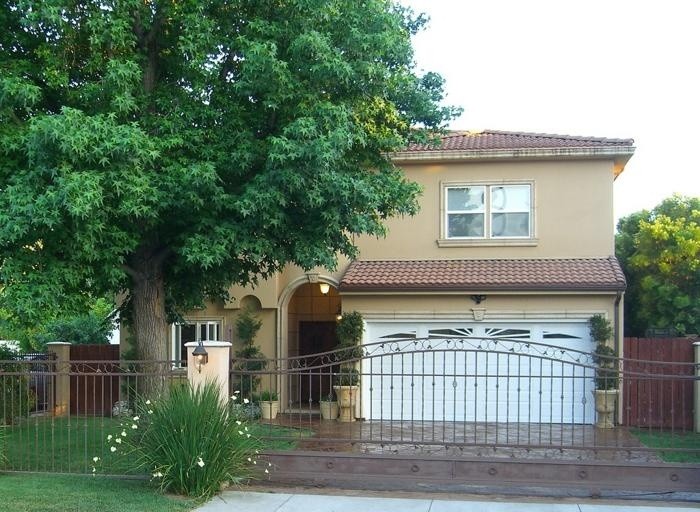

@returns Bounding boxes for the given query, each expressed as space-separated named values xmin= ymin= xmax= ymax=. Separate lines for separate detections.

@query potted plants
xmin=588 ymin=312 xmax=620 ymax=429
xmin=332 ymin=310 xmax=364 ymax=423
xmin=259 ymin=390 xmax=279 ymax=419
xmin=319 ymin=395 xmax=339 ymax=419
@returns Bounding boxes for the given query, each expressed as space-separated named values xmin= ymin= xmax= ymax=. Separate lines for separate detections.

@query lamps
xmin=192 ymin=338 xmax=208 ymax=373
xmin=335 ymin=304 xmax=342 ymax=323
xmin=319 ymin=283 xmax=330 ymax=295
